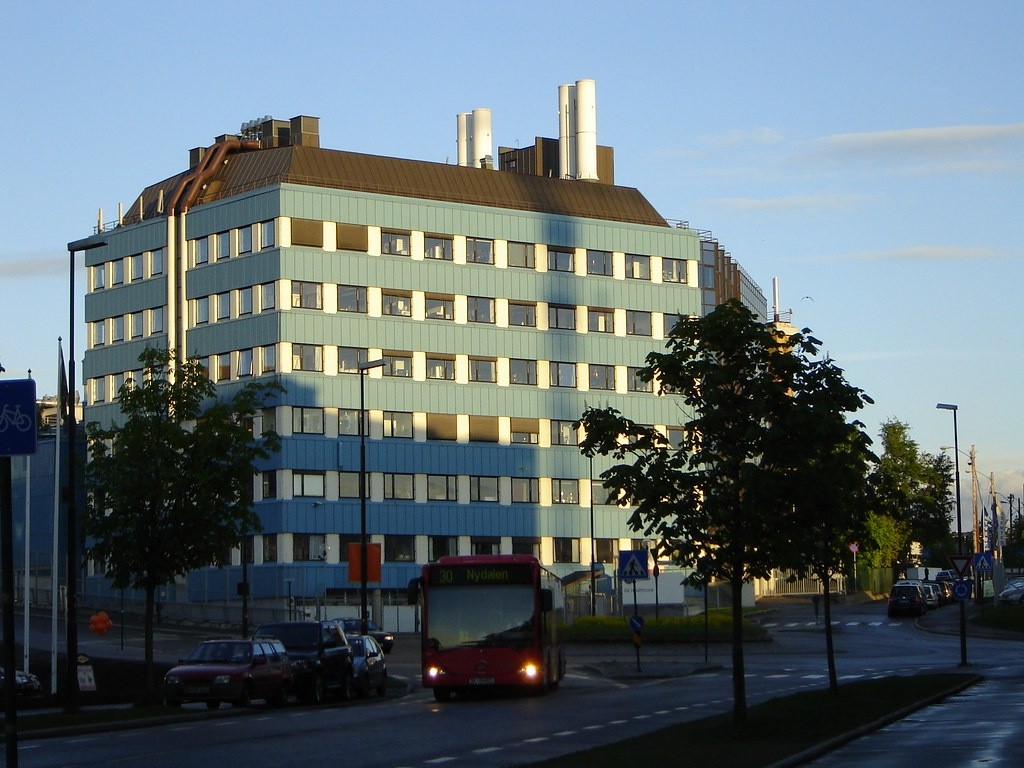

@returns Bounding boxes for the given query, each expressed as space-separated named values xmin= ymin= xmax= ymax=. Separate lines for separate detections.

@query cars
xmin=332 ymin=618 xmax=393 ymax=654
xmin=887 ymin=571 xmax=976 ymax=618
xmin=344 ymin=634 xmax=387 ymax=700
xmin=0 ymin=667 xmax=41 ymax=705
xmin=999 ymin=576 xmax=1024 ymax=604
xmin=162 ymin=636 xmax=296 ymax=714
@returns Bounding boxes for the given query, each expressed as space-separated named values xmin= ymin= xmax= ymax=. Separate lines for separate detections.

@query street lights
xmin=68 ymin=232 xmax=109 ymax=717
xmin=965 ymin=470 xmax=997 ymax=566
xmin=989 ymin=491 xmax=1013 ymax=573
xmin=585 ymin=453 xmax=595 ymax=616
xmin=936 ymin=402 xmax=972 ymax=667
xmin=360 ymin=358 xmax=388 ymax=637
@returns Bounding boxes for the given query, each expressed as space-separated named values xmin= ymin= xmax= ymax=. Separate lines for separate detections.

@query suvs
xmin=230 ymin=619 xmax=355 ymax=707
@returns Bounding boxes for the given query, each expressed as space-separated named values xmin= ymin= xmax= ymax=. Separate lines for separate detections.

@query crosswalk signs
xmin=617 ymin=548 xmax=651 ymax=581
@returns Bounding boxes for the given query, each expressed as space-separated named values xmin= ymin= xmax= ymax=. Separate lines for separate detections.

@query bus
xmin=407 ymin=555 xmax=564 ymax=701
